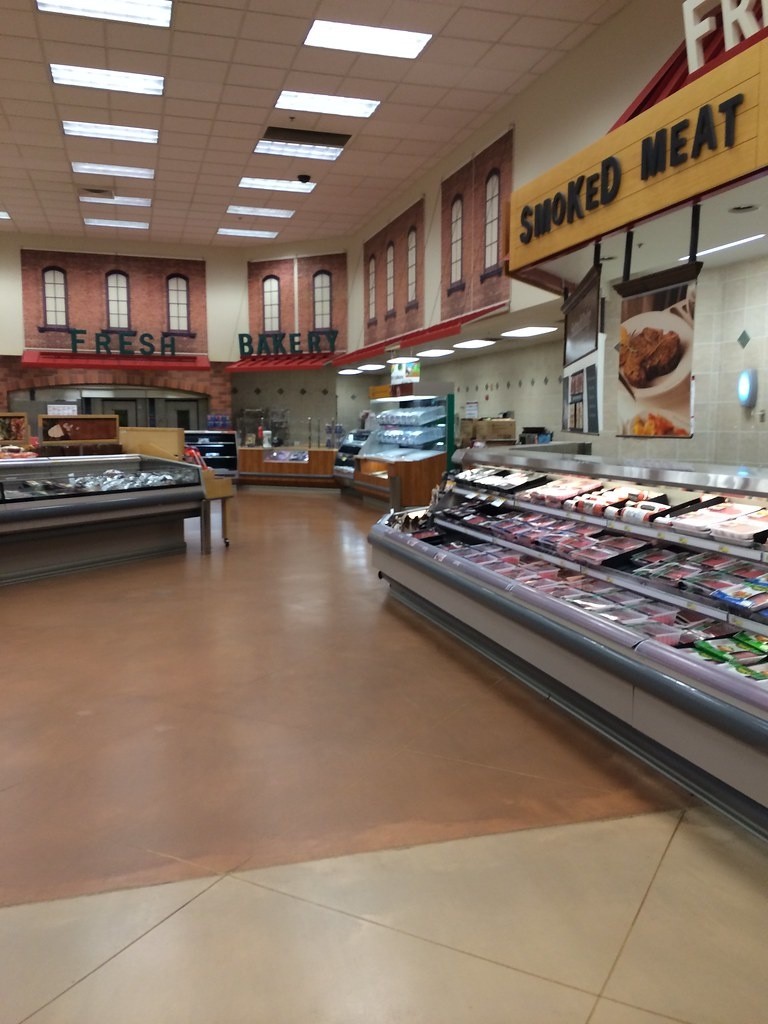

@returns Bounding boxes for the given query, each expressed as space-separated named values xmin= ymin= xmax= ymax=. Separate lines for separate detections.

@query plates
xmin=620 ymin=311 xmax=693 ymax=398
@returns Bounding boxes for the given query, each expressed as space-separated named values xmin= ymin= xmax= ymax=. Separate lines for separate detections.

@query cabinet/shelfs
xmin=358 ymin=393 xmax=456 ymax=462
xmin=332 ymin=428 xmax=373 ymax=480
xmin=183 ymin=430 xmax=239 ymax=479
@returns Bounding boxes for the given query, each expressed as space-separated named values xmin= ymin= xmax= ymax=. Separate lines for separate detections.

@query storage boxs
xmin=476 ymin=416 xmax=515 ymax=440
xmin=460 ymin=417 xmax=477 ymax=439
xmin=368 ymin=383 xmax=413 ymax=398
